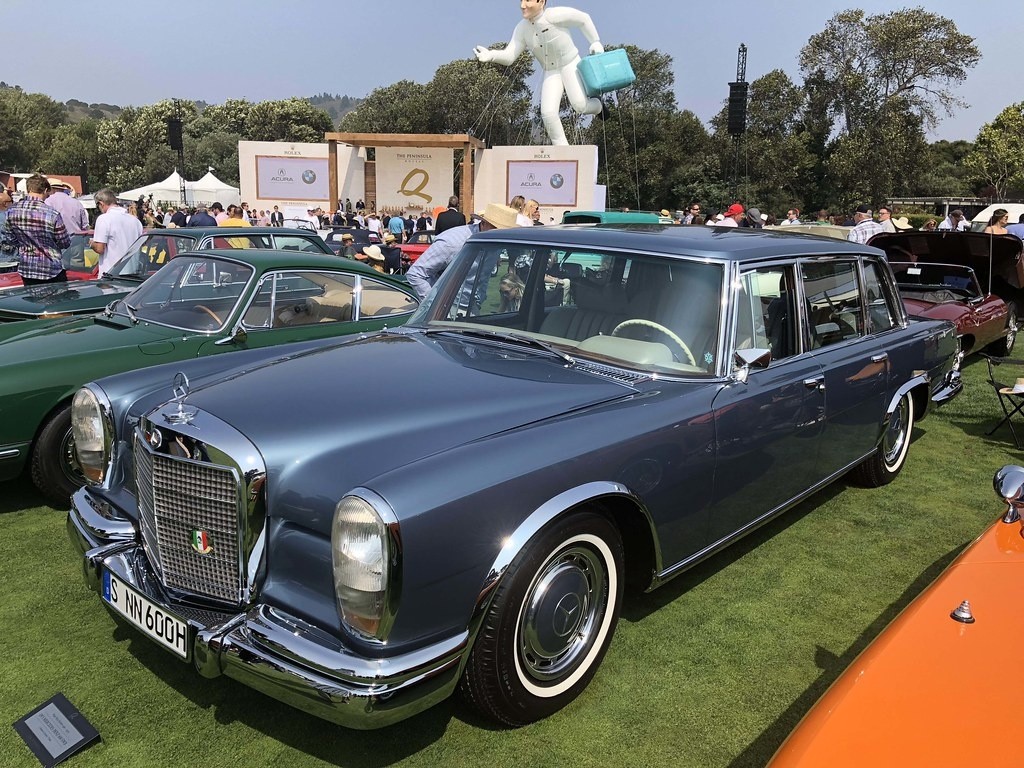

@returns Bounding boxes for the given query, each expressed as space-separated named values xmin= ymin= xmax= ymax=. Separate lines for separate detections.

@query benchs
xmin=274 ymin=295 xmax=404 ymax=328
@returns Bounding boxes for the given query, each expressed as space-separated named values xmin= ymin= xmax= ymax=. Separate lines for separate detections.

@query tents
xmin=120 ymin=171 xmax=240 ymax=206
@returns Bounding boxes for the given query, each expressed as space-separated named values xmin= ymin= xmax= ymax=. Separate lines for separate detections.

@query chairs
xmin=978 ymin=352 xmax=1024 ymax=451
xmin=538 ymin=274 xmax=719 ymax=372
xmin=378 ymin=245 xmax=411 ymax=276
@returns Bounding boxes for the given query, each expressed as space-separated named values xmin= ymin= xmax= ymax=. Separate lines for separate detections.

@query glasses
xmin=499 ymin=287 xmax=514 ymax=295
xmin=878 ymin=212 xmax=891 ymax=215
xmin=532 ymin=206 xmax=538 ymax=210
xmin=693 ymin=207 xmax=701 ymax=211
xmin=1003 ymin=216 xmax=1009 ymax=220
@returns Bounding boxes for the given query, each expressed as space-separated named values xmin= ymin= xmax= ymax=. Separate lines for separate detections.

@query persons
xmin=804 ymin=205 xmax=913 ymax=245
xmin=543 ymin=274 xmax=571 ymax=306
xmin=549 ymin=217 xmax=555 ymax=225
xmin=123 ymin=192 xmax=284 ymax=280
xmin=406 ymin=203 xmax=524 ymax=319
xmin=498 ymin=273 xmax=526 ymax=315
xmin=0 ymin=177 xmax=90 ymax=286
xmin=435 ymin=196 xmax=466 ymax=237
xmin=88 ymin=187 xmax=144 ymax=280
xmin=705 ymin=204 xmax=802 ymax=227
xmin=620 ymin=207 xmax=629 ymax=213
xmin=473 ymin=0 xmax=610 ymax=146
xmin=302 ymin=198 xmax=433 ymax=275
xmin=599 ymin=256 xmax=613 ymax=271
xmin=938 ymin=210 xmax=972 ymax=231
xmin=1005 ymin=213 xmax=1024 ymax=240
xmin=919 ymin=218 xmax=938 ymax=231
xmin=660 ymin=203 xmax=703 ymax=225
xmin=510 ymin=196 xmax=539 ymax=227
xmin=983 ymin=209 xmax=1008 ymax=235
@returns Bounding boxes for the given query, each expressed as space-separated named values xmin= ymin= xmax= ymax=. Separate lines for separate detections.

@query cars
xmin=266 ymin=216 xmax=438 ymax=268
xmin=0 ymin=226 xmax=339 ymax=322
xmin=67 ymin=222 xmax=966 ymax=727
xmin=764 ymin=464 xmax=1024 ymax=768
xmin=857 ymin=231 xmax=1024 ymax=371
xmin=968 ymin=203 xmax=1024 ymax=240
xmin=0 ymin=221 xmax=235 ymax=289
xmin=0 ymin=248 xmax=424 ymax=512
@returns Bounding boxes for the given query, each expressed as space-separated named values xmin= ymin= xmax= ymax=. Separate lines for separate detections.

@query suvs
xmin=516 ymin=210 xmax=663 ymax=292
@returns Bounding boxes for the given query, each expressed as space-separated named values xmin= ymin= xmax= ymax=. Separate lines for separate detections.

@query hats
xmin=363 ymin=245 xmax=385 ymax=260
xmin=659 ymin=209 xmax=671 ymax=218
xmin=47 ymin=178 xmax=69 ymax=189
xmin=951 ymin=210 xmax=965 ymax=220
xmin=385 ymin=235 xmax=398 ymax=242
xmin=724 ymin=204 xmax=743 ymax=217
xmin=748 ymin=208 xmax=762 ymax=222
xmin=342 ymin=234 xmax=353 ymax=240
xmin=850 ymin=205 xmax=872 ymax=217
xmin=470 ymin=203 xmax=522 ymax=229
xmin=892 ymin=217 xmax=913 ymax=229
